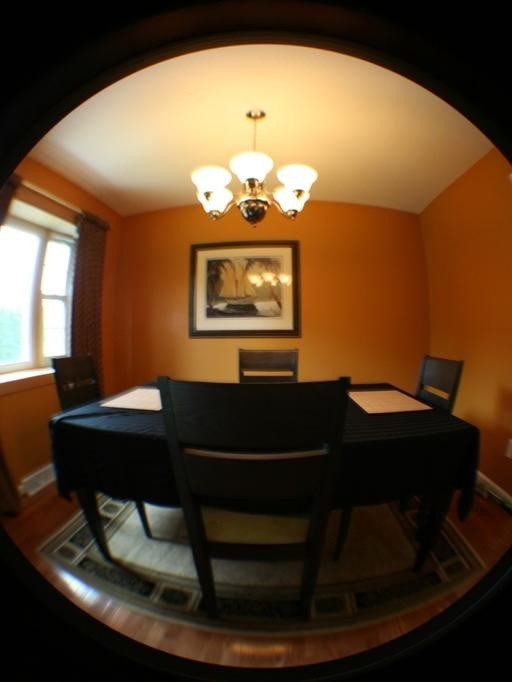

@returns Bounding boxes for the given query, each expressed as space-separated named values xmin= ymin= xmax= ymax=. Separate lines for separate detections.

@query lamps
xmin=189 ymin=107 xmax=317 ymax=223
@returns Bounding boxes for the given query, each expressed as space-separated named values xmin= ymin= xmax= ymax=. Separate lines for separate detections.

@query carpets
xmin=35 ymin=492 xmax=486 ymax=636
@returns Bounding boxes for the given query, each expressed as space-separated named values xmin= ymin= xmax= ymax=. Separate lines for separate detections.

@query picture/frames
xmin=188 ymin=239 xmax=301 ymax=338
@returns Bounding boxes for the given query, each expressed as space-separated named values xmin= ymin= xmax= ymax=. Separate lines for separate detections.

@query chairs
xmin=51 ymin=353 xmax=102 ymax=417
xmin=416 ymin=353 xmax=464 ymax=410
xmin=237 ymin=347 xmax=300 ymax=384
xmin=156 ymin=374 xmax=351 ymax=623
